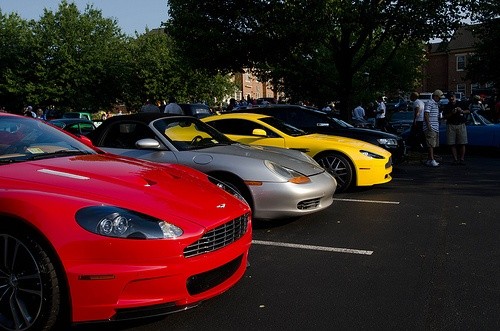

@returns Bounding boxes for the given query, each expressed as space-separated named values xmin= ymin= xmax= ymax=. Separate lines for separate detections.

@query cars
xmin=46 ymin=119 xmax=96 ymax=137
xmin=0 ymin=112 xmax=257 ymax=331
xmin=234 ymin=105 xmax=409 ymax=170
xmin=164 ymin=113 xmax=394 ymax=191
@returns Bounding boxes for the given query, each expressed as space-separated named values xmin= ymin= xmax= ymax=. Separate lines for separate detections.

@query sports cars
xmin=80 ymin=113 xmax=336 ymax=219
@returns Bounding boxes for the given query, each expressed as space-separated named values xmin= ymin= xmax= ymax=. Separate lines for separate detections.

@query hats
xmin=433 ymin=90 xmax=446 ymax=99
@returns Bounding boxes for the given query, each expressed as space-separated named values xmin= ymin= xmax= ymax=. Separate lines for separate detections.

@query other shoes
xmin=430 ymin=159 xmax=440 ymax=167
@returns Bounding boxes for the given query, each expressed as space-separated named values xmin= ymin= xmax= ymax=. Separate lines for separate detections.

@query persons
xmin=443 ymin=89 xmax=472 ymax=165
xmin=226 ymin=93 xmax=341 ymax=114
xmin=466 ymin=93 xmax=487 ymax=112
xmin=138 ymin=95 xmax=160 ymax=112
xmin=351 ymin=101 xmax=367 ymax=128
xmin=407 ymin=91 xmax=425 ymax=153
xmin=376 ymin=97 xmax=387 ymax=132
xmin=22 ymin=105 xmax=48 ymax=122
xmin=422 ymin=89 xmax=446 ymax=167
xmin=163 ymin=97 xmax=184 ymax=115
